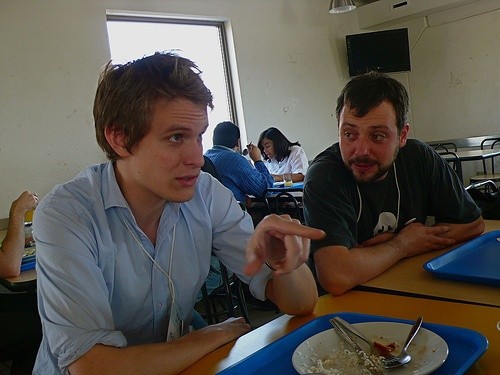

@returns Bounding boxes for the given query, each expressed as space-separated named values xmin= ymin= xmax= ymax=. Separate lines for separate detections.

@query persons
xmin=303 ymin=73 xmax=486 ymax=297
xmin=258 ymin=124 xmax=309 ymax=182
xmin=0 ymin=191 xmax=39 ymax=374
xmin=34 ymin=54 xmax=325 ymax=374
xmin=204 ymin=121 xmax=274 ymax=216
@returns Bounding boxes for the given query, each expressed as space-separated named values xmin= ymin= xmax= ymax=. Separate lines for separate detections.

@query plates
xmin=293 ymin=322 xmax=448 ymax=375
xmin=20 ymin=244 xmax=37 ymax=271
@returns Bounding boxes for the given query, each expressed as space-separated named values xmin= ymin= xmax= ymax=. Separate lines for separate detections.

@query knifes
xmin=328 ymin=319 xmax=383 ymax=374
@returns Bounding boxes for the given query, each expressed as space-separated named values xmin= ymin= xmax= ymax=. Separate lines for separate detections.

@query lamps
xmin=329 ymin=0 xmax=357 ymax=14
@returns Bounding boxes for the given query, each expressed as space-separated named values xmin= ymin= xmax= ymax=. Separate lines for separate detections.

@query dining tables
xmin=178 ymin=290 xmax=500 ymax=374
xmin=245 ymin=180 xmax=306 ymax=214
xmin=0 ymin=229 xmax=37 ymax=292
xmin=359 ymin=219 xmax=500 ymax=307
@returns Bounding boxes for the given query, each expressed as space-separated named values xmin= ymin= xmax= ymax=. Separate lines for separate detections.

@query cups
xmin=24 ymin=205 xmax=35 ymax=225
xmin=283 ymin=172 xmax=292 ymax=186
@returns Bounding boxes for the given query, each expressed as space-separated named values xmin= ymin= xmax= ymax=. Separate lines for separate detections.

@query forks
xmin=333 ymin=316 xmax=404 ymax=369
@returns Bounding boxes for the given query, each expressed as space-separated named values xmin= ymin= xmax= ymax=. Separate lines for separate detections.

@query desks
xmin=435 ymin=150 xmax=500 ymax=183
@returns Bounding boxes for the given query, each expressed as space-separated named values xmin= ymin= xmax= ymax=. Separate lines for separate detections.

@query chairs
xmin=428 ymin=137 xmax=500 ymax=194
xmin=201 ymin=260 xmax=253 ymax=330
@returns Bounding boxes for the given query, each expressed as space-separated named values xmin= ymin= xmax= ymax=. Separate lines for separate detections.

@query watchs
xmin=254 ymin=160 xmax=264 ymax=164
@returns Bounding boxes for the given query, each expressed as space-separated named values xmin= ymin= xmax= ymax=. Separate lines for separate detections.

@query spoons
xmin=386 ymin=316 xmax=425 ymax=370
